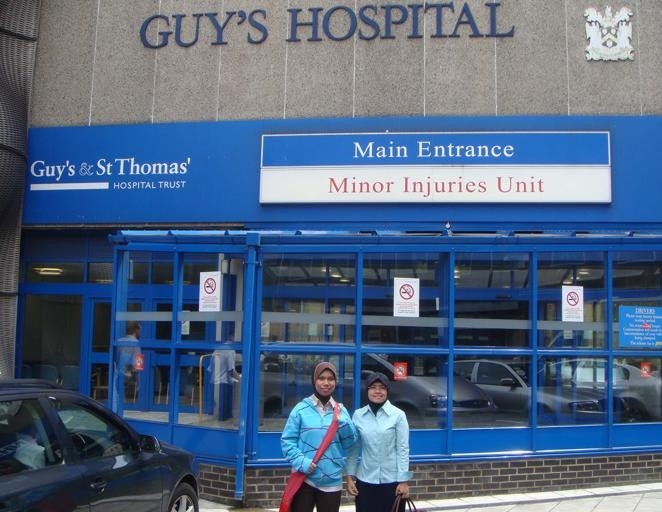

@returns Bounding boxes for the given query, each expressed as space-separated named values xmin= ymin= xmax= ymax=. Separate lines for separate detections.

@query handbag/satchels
xmin=279 ymin=471 xmax=306 ymax=512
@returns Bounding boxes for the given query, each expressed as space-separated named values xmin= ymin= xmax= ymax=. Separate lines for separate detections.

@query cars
xmin=0 ymin=379 xmax=199 ymax=511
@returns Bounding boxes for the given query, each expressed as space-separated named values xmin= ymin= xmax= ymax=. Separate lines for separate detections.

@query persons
xmin=204 ymin=334 xmax=242 ymax=421
xmin=342 ymin=371 xmax=415 ymax=511
xmin=280 ymin=360 xmax=359 ymax=512
xmin=113 ymin=319 xmax=144 ymax=399
